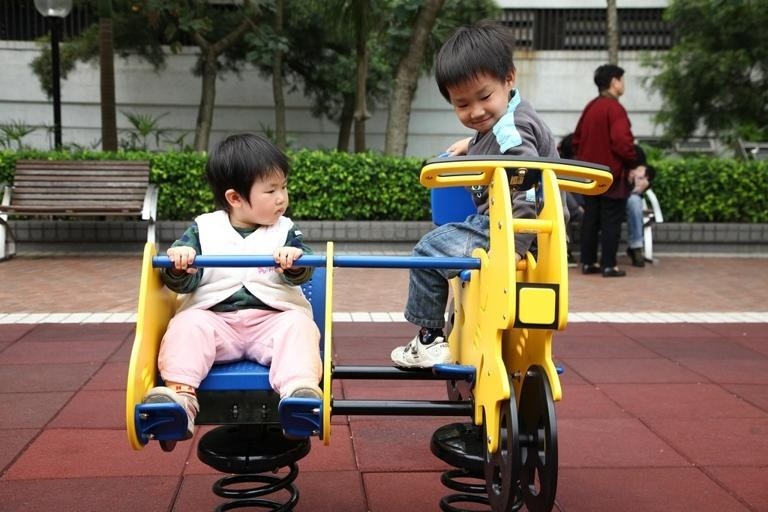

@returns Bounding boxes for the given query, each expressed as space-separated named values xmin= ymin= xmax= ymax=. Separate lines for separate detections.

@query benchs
xmin=564 ymin=183 xmax=666 ymax=263
xmin=0 ymin=159 xmax=163 ymax=261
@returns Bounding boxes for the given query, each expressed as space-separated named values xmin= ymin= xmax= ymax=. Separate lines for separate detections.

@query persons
xmin=143 ymin=131 xmax=324 ymax=442
xmin=557 ymin=64 xmax=656 ymax=278
xmin=389 ymin=16 xmax=572 ymax=368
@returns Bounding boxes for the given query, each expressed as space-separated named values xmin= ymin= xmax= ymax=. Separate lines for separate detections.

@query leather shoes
xmin=583 ymin=264 xmax=603 ymax=275
xmin=628 ymin=247 xmax=646 ymax=267
xmin=604 ymin=267 xmax=626 ymax=277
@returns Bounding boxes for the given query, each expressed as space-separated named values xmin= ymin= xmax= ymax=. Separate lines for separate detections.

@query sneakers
xmin=282 ymin=383 xmax=324 ymax=442
xmin=142 ymin=387 xmax=200 ymax=440
xmin=390 ymin=335 xmax=450 ymax=369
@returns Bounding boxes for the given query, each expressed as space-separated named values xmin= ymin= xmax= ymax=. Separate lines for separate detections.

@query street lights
xmin=33 ymin=1 xmax=75 ymax=149
